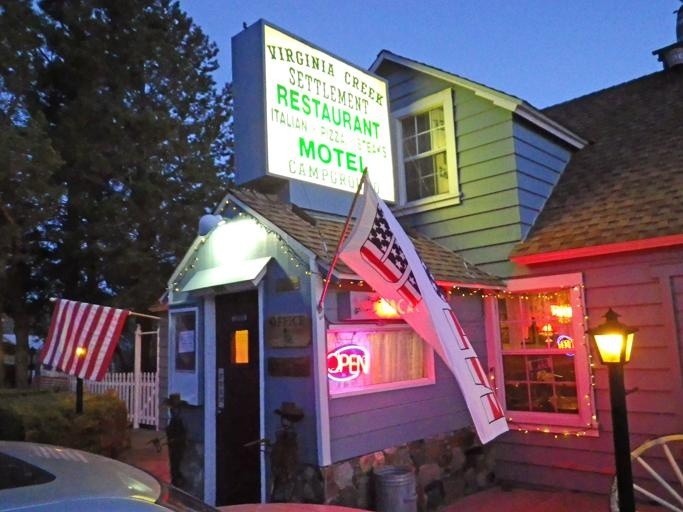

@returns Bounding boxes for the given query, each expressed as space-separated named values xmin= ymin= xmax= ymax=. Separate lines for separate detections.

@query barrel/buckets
xmin=370 ymin=465 xmax=419 ymax=512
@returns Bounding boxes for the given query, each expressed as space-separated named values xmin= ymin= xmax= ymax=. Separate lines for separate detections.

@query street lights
xmin=586 ymin=306 xmax=641 ymax=512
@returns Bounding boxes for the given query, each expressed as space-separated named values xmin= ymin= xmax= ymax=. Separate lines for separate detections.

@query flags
xmin=38 ymin=298 xmax=128 ymax=382
xmin=338 ymin=173 xmax=510 ymax=444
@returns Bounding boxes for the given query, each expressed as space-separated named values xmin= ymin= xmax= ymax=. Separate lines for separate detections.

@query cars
xmin=0 ymin=439 xmax=379 ymax=511
